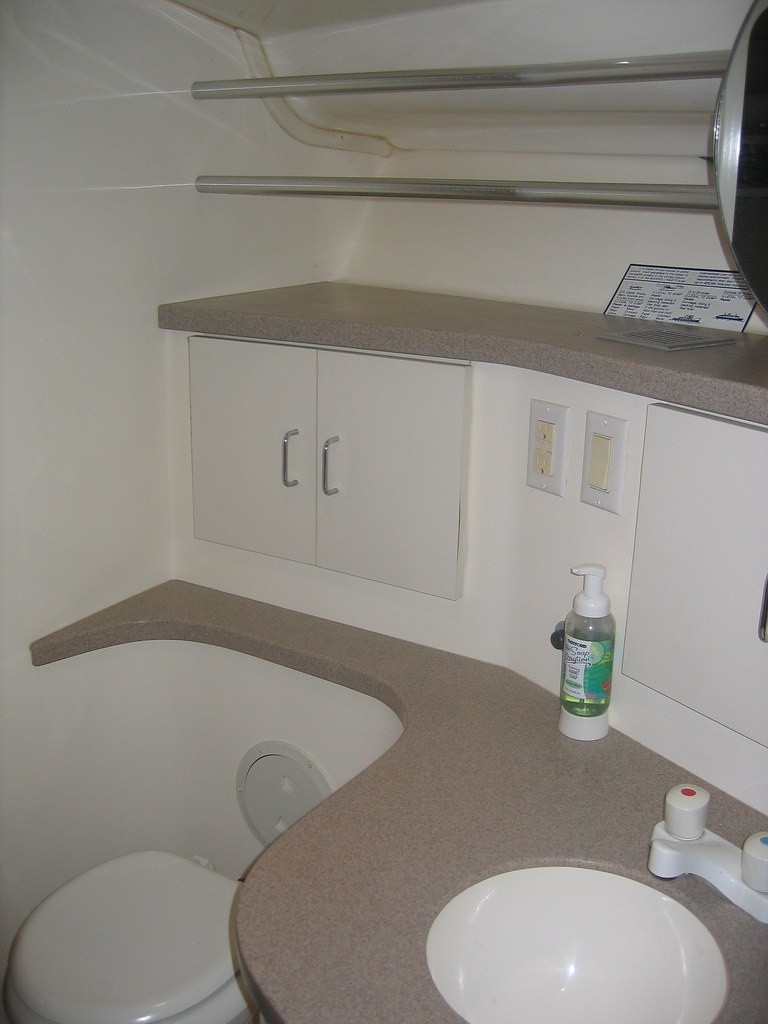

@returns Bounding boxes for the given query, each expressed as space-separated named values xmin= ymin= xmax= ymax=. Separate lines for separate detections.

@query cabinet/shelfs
xmin=621 ymin=402 xmax=768 ymax=745
xmin=188 ymin=336 xmax=473 ymax=603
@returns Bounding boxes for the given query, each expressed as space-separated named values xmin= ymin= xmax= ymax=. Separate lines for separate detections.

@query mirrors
xmin=707 ymin=0 xmax=768 ymax=330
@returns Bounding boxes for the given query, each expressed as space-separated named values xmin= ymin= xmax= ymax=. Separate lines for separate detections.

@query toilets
xmin=0 ymin=849 xmax=256 ymax=1024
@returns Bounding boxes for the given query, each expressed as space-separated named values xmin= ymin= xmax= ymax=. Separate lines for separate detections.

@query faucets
xmin=647 ymin=841 xmax=728 ymax=880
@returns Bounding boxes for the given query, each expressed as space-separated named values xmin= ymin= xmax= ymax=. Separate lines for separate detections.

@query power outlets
xmin=525 ymin=399 xmax=570 ymax=499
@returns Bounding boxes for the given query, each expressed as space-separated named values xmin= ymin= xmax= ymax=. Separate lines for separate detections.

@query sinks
xmin=425 ymin=865 xmax=728 ymax=1024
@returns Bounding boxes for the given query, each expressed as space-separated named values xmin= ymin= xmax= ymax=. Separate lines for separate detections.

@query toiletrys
xmin=556 ymin=563 xmax=617 ymax=741
